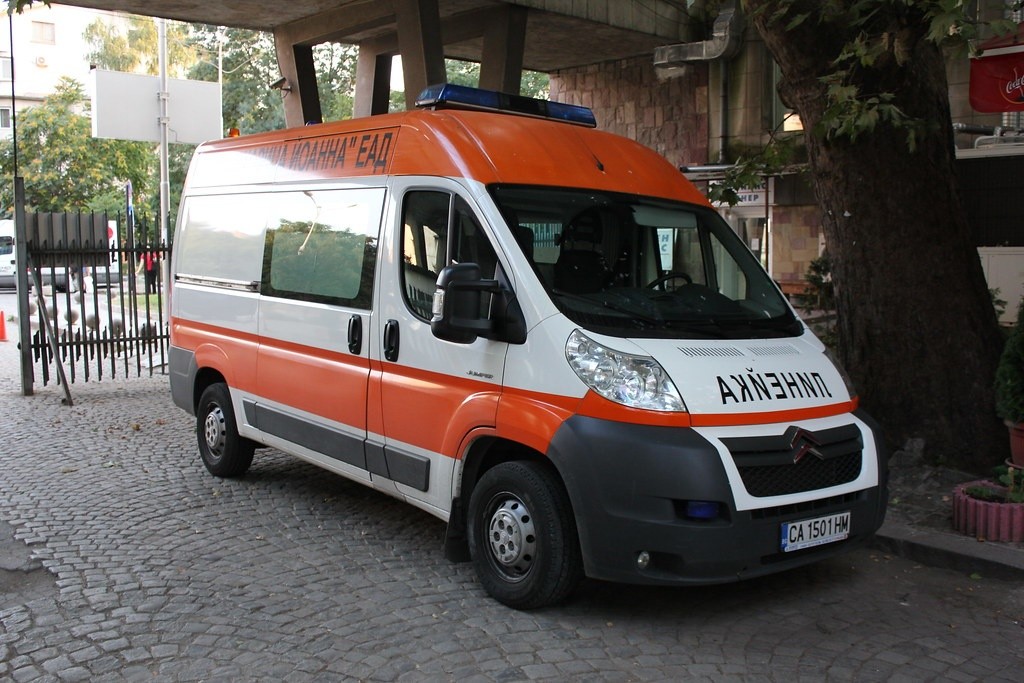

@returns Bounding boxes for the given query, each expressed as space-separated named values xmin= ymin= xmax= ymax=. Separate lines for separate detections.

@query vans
xmin=167 ymin=85 xmax=896 ymax=620
xmin=1 ymin=217 xmax=82 ymax=296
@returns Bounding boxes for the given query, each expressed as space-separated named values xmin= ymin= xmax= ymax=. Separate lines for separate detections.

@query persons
xmin=69 ymin=265 xmax=90 ymax=292
xmin=137 ymin=251 xmax=158 ymax=294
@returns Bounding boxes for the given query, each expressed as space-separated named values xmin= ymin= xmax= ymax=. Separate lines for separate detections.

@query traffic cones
xmin=0 ymin=310 xmax=11 ymax=342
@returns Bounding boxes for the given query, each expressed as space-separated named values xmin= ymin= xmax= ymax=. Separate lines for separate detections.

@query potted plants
xmin=993 ymin=291 xmax=1023 ymax=468
